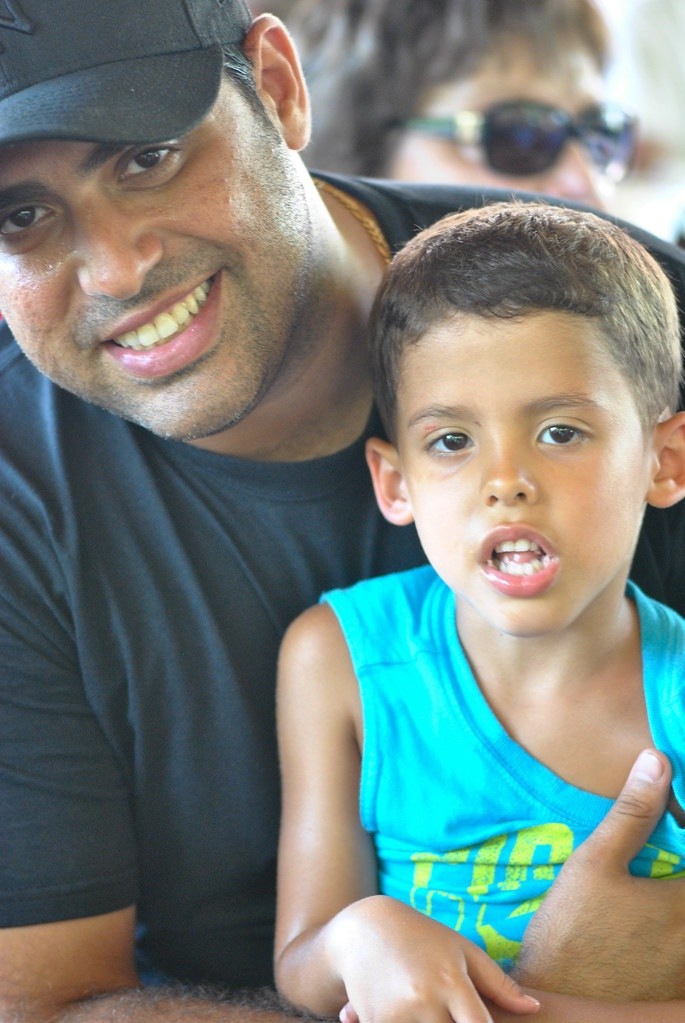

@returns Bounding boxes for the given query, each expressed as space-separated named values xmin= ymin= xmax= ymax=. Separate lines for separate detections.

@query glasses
xmin=383 ymin=99 xmax=638 ymax=186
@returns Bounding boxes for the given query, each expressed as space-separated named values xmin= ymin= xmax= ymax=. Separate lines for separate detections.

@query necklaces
xmin=312 ymin=178 xmax=392 ymax=268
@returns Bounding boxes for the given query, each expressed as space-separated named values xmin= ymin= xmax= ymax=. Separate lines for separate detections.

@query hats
xmin=0 ymin=0 xmax=251 ymax=144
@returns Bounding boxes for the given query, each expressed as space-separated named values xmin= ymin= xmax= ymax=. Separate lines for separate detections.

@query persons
xmin=0 ymin=0 xmax=685 ymax=1023
xmin=251 ymin=0 xmax=639 ymax=212
xmin=272 ymin=201 xmax=685 ymax=1023
